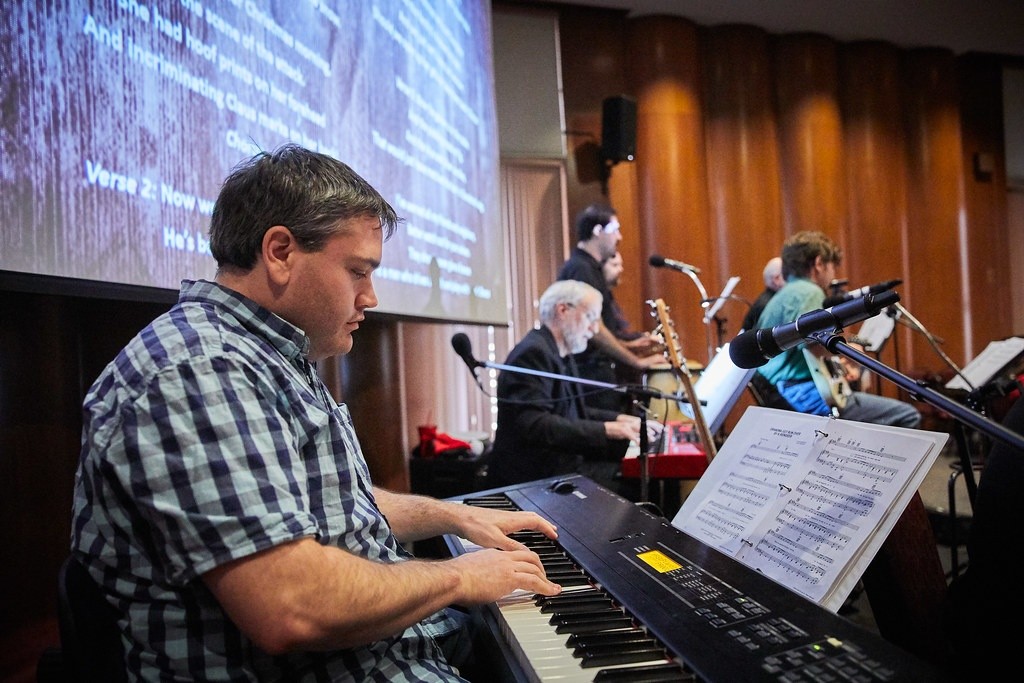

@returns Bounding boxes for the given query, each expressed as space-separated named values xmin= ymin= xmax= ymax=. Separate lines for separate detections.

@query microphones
xmin=648 ymin=254 xmax=700 ymax=274
xmin=452 ymin=333 xmax=482 ymax=388
xmin=729 ymin=289 xmax=901 ymax=369
xmin=822 ymin=278 xmax=903 ymax=310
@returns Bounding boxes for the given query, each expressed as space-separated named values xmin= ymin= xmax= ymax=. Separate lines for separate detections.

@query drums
xmin=640 ymin=357 xmax=704 ymax=424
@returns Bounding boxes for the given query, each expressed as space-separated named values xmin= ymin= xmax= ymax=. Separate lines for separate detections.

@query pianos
xmin=441 ymin=472 xmax=924 ymax=683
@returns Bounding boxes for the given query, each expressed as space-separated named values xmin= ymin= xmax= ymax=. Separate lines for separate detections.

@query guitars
xmin=798 ymin=332 xmax=872 ymax=410
xmin=645 ymin=296 xmax=720 ymax=466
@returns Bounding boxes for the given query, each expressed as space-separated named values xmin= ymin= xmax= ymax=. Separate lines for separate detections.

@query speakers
xmin=603 ymin=94 xmax=638 ymax=161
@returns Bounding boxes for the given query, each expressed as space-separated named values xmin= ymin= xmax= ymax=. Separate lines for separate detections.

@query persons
xmin=489 ymin=280 xmax=680 ymax=520
xmin=556 ymin=203 xmax=660 ymax=413
xmin=741 ymin=232 xmax=923 ymax=432
xmin=71 ymin=143 xmax=561 ymax=682
